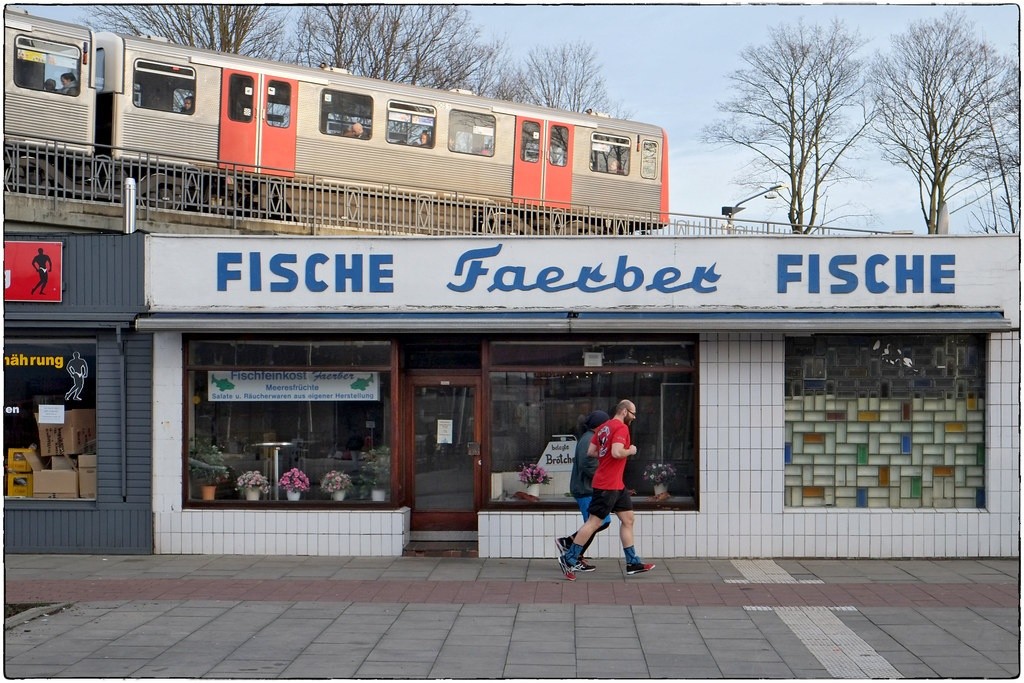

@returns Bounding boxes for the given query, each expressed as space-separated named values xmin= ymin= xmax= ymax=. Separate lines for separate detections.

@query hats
xmin=584 ymin=410 xmax=610 ymax=429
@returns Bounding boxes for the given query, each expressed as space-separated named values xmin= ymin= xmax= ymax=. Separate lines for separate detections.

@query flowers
xmin=235 ymin=470 xmax=272 ymax=494
xmin=278 ymin=467 xmax=311 ymax=493
xmin=518 ymin=463 xmax=554 ymax=488
xmin=318 ymin=469 xmax=355 ymax=500
xmin=188 ymin=444 xmax=236 ymax=486
xmin=640 ymin=462 xmax=679 ymax=488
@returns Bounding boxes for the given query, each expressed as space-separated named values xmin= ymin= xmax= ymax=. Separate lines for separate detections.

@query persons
xmin=42 ymin=73 xmax=80 ymax=97
xmin=557 ymin=399 xmax=656 ymax=581
xmin=412 ymin=132 xmax=433 ymax=148
xmin=344 ymin=122 xmax=370 ymax=140
xmin=449 ymin=132 xmax=455 ymax=150
xmin=606 ymin=157 xmax=624 ymax=174
xmin=555 ymin=410 xmax=611 ymax=572
xmin=180 ymin=96 xmax=195 ymax=115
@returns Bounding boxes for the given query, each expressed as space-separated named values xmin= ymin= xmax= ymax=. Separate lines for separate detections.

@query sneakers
xmin=555 ymin=537 xmax=571 ymax=555
xmin=573 ymin=556 xmax=596 ymax=571
xmin=626 ymin=562 xmax=656 ymax=574
xmin=557 ymin=555 xmax=576 ymax=581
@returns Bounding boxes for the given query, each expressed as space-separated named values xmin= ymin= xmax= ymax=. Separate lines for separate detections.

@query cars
xmin=296 ymin=440 xmax=376 ymax=485
xmin=189 ymin=456 xmax=239 ymax=497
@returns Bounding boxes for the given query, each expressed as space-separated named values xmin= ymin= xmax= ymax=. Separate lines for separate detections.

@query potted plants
xmin=357 ymin=445 xmax=391 ymax=502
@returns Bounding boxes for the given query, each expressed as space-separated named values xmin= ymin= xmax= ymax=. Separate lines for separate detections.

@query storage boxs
xmin=7 ymin=473 xmax=32 ymax=496
xmin=34 ymin=409 xmax=96 ymax=457
xmin=78 ymin=455 xmax=96 ymax=498
xmin=23 ymin=452 xmax=79 ymax=498
xmin=8 ymin=448 xmax=32 ymax=473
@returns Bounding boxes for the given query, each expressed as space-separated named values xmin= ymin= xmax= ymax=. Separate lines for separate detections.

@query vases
xmin=287 ymin=490 xmax=301 ymax=501
xmin=527 ymin=484 xmax=540 ymax=497
xmin=200 ymin=485 xmax=216 ymax=500
xmin=653 ymin=483 xmax=668 ymax=495
xmin=332 ymin=490 xmax=346 ymax=501
xmin=244 ymin=485 xmax=260 ymax=501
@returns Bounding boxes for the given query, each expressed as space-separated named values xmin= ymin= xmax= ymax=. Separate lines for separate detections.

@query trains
xmin=3 ymin=8 xmax=669 ymax=237
